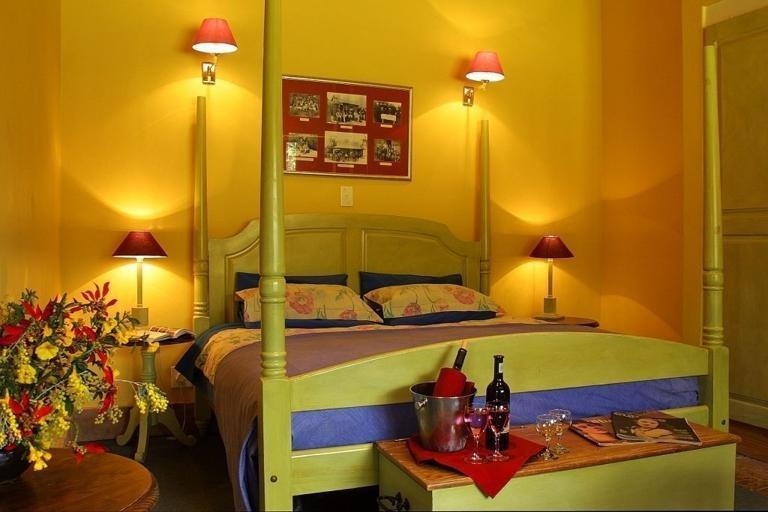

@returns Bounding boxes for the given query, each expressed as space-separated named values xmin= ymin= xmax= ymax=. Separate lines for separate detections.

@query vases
xmin=1 ymin=442 xmax=40 ymax=487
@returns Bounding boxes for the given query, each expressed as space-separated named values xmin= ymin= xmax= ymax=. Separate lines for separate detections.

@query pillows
xmin=356 ymin=271 xmax=464 ymax=302
xmin=235 ymin=269 xmax=349 ymax=323
xmin=235 ymin=282 xmax=385 ymax=324
xmin=362 ymin=282 xmax=509 ymax=320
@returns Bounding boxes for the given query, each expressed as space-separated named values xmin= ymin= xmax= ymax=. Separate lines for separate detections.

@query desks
xmin=536 ymin=317 xmax=600 ymax=329
xmin=103 ymin=327 xmax=197 ymax=463
xmin=1 ymin=446 xmax=162 ymax=511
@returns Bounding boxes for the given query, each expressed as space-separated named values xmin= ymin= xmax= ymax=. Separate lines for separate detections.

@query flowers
xmin=0 ymin=280 xmax=171 ymax=473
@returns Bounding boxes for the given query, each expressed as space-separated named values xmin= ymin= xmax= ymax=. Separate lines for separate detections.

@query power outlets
xmin=340 ymin=185 xmax=354 ymax=208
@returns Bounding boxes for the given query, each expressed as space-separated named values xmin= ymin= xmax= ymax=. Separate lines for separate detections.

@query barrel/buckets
xmin=410 ymin=382 xmax=478 ymax=453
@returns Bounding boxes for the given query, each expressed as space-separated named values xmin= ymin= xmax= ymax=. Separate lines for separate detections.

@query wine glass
xmin=488 ymin=400 xmax=512 ymax=461
xmin=461 ymin=402 xmax=491 ymax=466
xmin=535 ymin=407 xmax=574 ymax=460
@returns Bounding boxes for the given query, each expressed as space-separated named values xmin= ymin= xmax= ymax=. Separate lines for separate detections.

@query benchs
xmin=373 ymin=409 xmax=744 ymax=511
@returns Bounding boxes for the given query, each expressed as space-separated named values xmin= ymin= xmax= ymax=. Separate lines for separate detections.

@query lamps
xmin=190 ymin=16 xmax=239 ymax=86
xmin=528 ymin=235 xmax=576 ymax=322
xmin=462 ymin=51 xmax=506 ymax=107
xmin=111 ymin=230 xmax=169 ymax=328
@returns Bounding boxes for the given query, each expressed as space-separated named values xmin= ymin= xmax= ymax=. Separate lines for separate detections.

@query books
xmin=564 ymin=413 xmax=649 ymax=447
xmin=611 ymin=406 xmax=704 ymax=446
xmin=132 ymin=322 xmax=197 ymax=341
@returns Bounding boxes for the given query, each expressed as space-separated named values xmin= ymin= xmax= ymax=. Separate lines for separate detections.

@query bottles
xmin=485 ymin=355 xmax=510 ymax=450
xmin=432 ymin=347 xmax=468 ymax=397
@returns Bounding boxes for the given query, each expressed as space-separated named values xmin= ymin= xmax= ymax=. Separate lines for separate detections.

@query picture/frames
xmin=281 ymin=74 xmax=414 ymax=182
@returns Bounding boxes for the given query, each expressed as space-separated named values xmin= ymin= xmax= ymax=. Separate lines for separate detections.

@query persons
xmin=328 ymin=100 xmax=366 ymax=125
xmin=574 ymin=418 xmax=621 ymax=444
xmin=633 ymin=415 xmax=672 ymax=436
xmin=327 ymin=138 xmax=366 ymax=162
xmin=375 ymin=142 xmax=400 ymax=161
xmin=289 ymin=136 xmax=314 ymax=156
xmin=374 ymin=101 xmax=400 ymax=122
xmin=290 ymin=95 xmax=319 ymax=117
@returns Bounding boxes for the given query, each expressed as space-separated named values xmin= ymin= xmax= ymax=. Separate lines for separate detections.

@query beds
xmin=174 ymin=1 xmax=733 ymax=510
